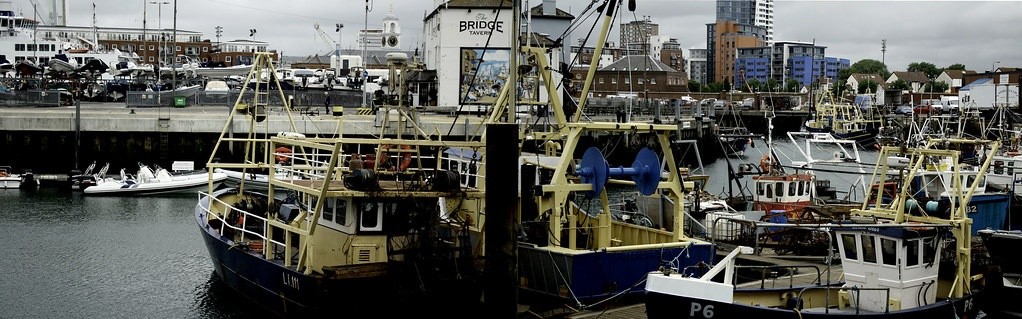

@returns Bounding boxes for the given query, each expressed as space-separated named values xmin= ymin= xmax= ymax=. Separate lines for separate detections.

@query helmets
xmin=324 ymin=92 xmax=328 ymax=96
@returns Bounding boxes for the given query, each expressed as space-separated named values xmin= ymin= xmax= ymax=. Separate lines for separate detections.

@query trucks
xmin=855 ymin=95 xmax=872 ymax=112
xmin=941 ymin=96 xmax=959 ymax=114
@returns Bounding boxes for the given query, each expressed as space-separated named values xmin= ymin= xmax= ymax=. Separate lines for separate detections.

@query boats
xmin=85 ymin=163 xmax=227 ymax=198
xmin=194 ymin=1 xmax=1022 ymax=319
xmin=0 ymin=166 xmax=95 ymax=189
xmin=214 ymin=168 xmax=304 ymax=187
xmin=0 ymin=10 xmax=200 ymax=106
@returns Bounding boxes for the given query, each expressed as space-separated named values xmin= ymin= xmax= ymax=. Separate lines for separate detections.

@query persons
xmin=14 ymin=81 xmax=85 ymax=104
xmin=87 ymin=85 xmax=93 ymax=102
xmin=616 ymin=106 xmax=626 ymax=123
xmin=324 ymin=92 xmax=331 ymax=115
xmin=654 ymin=116 xmax=659 ymax=124
xmin=107 ymin=75 xmax=158 ymax=104
xmin=0 ymin=81 xmax=12 ymax=104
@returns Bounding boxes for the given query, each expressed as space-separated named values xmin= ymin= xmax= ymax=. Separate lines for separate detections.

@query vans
xmin=921 ymin=99 xmax=944 ymax=116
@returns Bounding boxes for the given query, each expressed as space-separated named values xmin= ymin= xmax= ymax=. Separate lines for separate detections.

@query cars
xmin=745 ymin=101 xmax=753 ymax=107
xmin=681 ymin=96 xmax=699 ymax=103
xmin=914 ymin=104 xmax=936 ymax=115
xmin=896 ymin=106 xmax=915 ymax=115
xmin=714 ymin=99 xmax=726 ymax=109
xmin=732 ymin=101 xmax=741 ymax=108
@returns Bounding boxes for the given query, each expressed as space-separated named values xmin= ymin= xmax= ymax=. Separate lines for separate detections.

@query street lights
xmin=787 ymin=69 xmax=790 ymax=91
xmin=151 ymin=1 xmax=170 ymax=106
xmin=703 ymin=73 xmax=706 ymax=84
xmin=993 ymin=62 xmax=1000 ymax=72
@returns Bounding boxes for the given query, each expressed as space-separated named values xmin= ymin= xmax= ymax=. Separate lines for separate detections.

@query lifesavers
xmin=276 ymin=150 xmax=290 ymax=162
xmin=760 ymin=155 xmax=774 ymax=171
xmin=381 ymin=144 xmax=411 ymax=171
